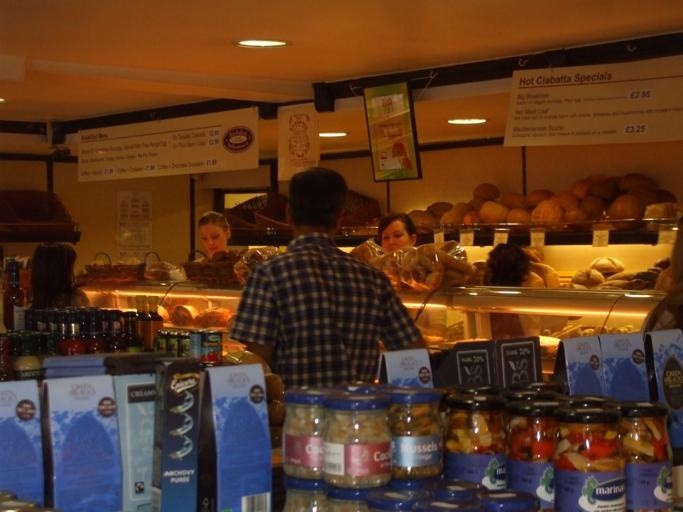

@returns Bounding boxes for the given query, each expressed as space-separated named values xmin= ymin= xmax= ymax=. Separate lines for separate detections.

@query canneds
xmin=153 ymin=330 xmax=222 ymax=366
xmin=281 ymin=380 xmax=674 ymax=511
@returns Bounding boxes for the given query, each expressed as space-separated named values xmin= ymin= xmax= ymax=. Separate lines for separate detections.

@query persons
xmin=642 ymin=215 xmax=682 ymax=330
xmin=230 ymin=169 xmax=431 ymax=386
xmin=29 ymin=240 xmax=89 ymax=308
xmin=193 ymin=211 xmax=233 ymax=262
xmin=475 ymin=243 xmax=541 ymax=341
xmin=377 ymin=213 xmax=448 ymax=339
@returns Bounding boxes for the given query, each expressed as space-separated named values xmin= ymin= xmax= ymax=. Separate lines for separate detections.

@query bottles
xmin=25 ymin=306 xmax=191 ymax=356
xmin=3 ymin=262 xmax=26 ymax=328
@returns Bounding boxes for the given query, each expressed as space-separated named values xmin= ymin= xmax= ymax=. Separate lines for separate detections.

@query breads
xmin=350 ymin=241 xmax=673 ymax=290
xmin=541 ymin=324 xmax=639 ymax=341
xmin=407 ymin=174 xmax=683 ymax=225
xmin=204 ymin=249 xmax=266 ymax=287
xmin=155 ymin=306 xmax=238 ymax=332
